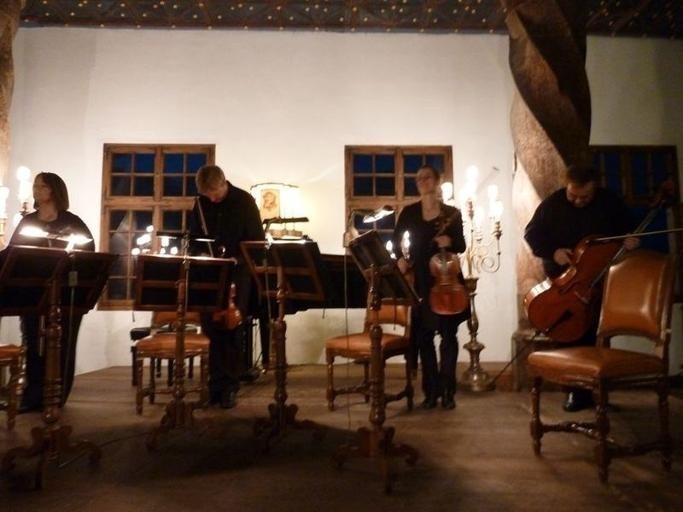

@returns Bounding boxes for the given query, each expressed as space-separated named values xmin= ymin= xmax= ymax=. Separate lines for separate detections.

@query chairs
xmin=325 ymin=292 xmax=411 ymax=409
xmin=524 ymin=249 xmax=681 ymax=491
xmin=134 ymin=308 xmax=212 ymax=415
xmin=1 ymin=343 xmax=23 ymax=428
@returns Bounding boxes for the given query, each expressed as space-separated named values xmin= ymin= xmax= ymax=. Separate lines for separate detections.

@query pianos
xmin=239 ymin=253 xmax=409 ymax=381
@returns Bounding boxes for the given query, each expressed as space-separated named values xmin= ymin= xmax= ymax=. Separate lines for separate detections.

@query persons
xmin=188 ymin=165 xmax=265 ymax=408
xmin=390 ymin=163 xmax=471 ymax=409
xmin=523 ymin=166 xmax=641 ymax=412
xmin=0 ymin=172 xmax=95 ymax=414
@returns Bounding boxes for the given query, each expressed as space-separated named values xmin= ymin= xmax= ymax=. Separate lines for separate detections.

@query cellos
xmin=523 ymin=180 xmax=678 ymax=342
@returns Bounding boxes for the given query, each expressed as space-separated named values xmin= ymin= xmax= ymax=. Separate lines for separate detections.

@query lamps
xmin=259 ymin=212 xmax=309 ymax=243
xmin=385 ymin=180 xmax=501 ymax=395
xmin=153 ymin=226 xmax=220 ymax=247
xmin=0 ymin=165 xmax=31 ymax=248
xmin=337 ymin=203 xmax=394 ymax=245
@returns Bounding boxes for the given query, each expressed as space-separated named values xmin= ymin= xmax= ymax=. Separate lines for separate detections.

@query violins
xmin=211 ymin=257 xmax=243 ymax=331
xmin=428 ymin=209 xmax=469 ymax=315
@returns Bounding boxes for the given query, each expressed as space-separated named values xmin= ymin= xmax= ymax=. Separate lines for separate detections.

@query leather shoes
xmin=224 ymin=390 xmax=237 ymax=409
xmin=563 ymin=392 xmax=584 ymax=410
xmin=422 ymin=392 xmax=440 ymax=410
xmin=444 ymin=390 xmax=455 ymax=410
